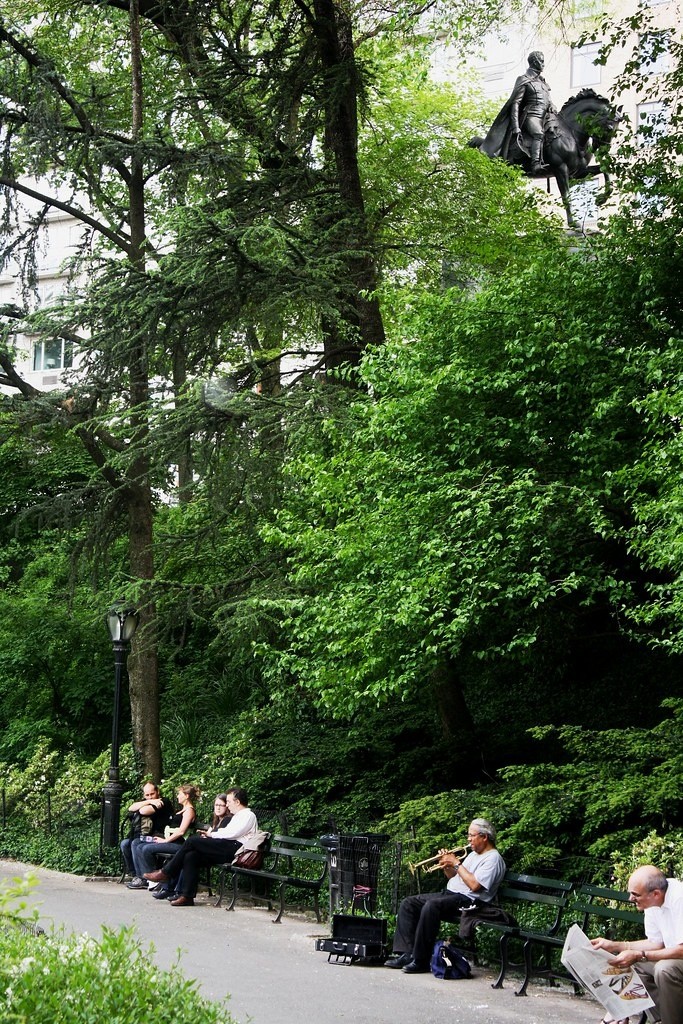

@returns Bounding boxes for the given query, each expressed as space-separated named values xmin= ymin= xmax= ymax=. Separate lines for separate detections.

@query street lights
xmin=98 ymin=600 xmax=140 ymax=847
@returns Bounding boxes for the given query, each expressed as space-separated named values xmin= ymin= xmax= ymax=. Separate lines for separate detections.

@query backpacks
xmin=129 ymin=810 xmax=152 ymax=841
xmin=430 ymin=940 xmax=472 ymax=980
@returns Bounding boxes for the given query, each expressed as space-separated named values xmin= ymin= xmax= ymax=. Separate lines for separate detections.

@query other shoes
xmin=126 ymin=876 xmax=149 ymax=889
xmin=152 ymin=889 xmax=176 ymax=899
xmin=167 ymin=893 xmax=180 ymax=901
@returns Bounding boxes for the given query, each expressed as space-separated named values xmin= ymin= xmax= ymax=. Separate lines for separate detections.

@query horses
xmin=464 ymin=89 xmax=619 ymax=228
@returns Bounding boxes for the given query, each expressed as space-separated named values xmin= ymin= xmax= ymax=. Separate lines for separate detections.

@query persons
xmin=121 ymin=781 xmax=174 ymax=889
xmin=385 ymin=818 xmax=506 ymax=974
xmin=589 ymin=865 xmax=683 ymax=1024
xmin=137 ymin=785 xmax=201 ymax=891
xmin=144 ymin=787 xmax=258 ymax=906
xmin=510 ymin=50 xmax=558 ymax=173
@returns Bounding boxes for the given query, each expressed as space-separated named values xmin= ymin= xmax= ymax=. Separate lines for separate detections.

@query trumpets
xmin=407 ymin=843 xmax=472 ymax=875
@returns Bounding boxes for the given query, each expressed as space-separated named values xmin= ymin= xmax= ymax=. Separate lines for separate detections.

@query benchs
xmin=514 ymin=884 xmax=646 ymax=995
xmin=411 ymin=870 xmax=574 ymax=992
xmin=120 ymin=811 xmax=330 ymax=925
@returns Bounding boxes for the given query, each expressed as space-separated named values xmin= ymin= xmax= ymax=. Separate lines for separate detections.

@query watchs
xmin=454 ymin=862 xmax=461 ymax=871
xmin=640 ymin=951 xmax=648 ymax=962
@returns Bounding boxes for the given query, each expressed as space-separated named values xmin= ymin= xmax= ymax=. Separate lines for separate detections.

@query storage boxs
xmin=315 ymin=914 xmax=387 ymax=966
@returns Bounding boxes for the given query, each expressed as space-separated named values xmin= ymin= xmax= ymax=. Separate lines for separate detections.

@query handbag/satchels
xmin=236 ymin=832 xmax=272 ymax=870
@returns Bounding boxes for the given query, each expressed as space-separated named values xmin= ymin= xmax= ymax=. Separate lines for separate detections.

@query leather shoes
xmin=170 ymin=896 xmax=195 ymax=906
xmin=143 ymin=870 xmax=168 ymax=882
xmin=402 ymin=960 xmax=431 ymax=973
xmin=384 ymin=953 xmax=414 ymax=968
